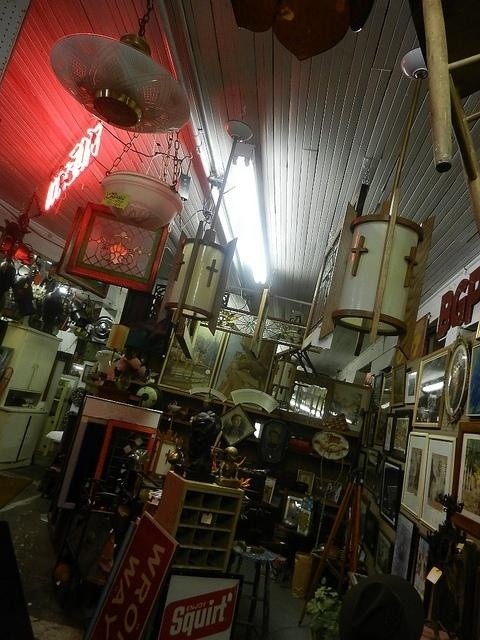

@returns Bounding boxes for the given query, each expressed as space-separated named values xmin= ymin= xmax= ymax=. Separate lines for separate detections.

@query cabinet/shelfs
xmin=0 ymin=322 xmax=63 ymax=409
xmin=155 ymin=468 xmax=244 ymax=577
xmin=0 ymin=406 xmax=46 ymax=468
xmin=50 ymin=394 xmax=165 ymax=516
xmin=152 ymin=437 xmax=177 ymax=476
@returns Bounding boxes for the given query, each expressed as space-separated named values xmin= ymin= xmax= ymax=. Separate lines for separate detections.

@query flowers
xmin=104 ymin=344 xmax=156 ymax=383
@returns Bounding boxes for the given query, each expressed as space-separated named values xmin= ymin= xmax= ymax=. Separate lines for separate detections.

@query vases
xmin=117 ymin=372 xmax=132 ymax=390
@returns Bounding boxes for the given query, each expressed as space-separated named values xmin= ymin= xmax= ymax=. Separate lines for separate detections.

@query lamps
xmin=316 ymin=46 xmax=440 ymax=360
xmin=0 ymin=187 xmax=90 ymax=327
xmin=105 ymin=322 xmax=131 ymax=365
xmin=231 ymin=138 xmax=273 ymax=285
xmin=231 ymin=0 xmax=377 ymax=64
xmin=156 ymin=116 xmax=254 ymax=338
xmin=49 ymin=0 xmax=192 ymax=134
xmin=164 ymin=398 xmax=182 ymax=440
xmin=269 ymin=345 xmax=299 ymax=409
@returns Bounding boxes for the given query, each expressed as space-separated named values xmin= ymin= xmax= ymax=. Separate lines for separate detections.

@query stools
xmin=230 ymin=544 xmax=275 ymax=636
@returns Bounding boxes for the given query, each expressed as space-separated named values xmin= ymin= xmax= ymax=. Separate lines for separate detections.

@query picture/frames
xmin=56 ymin=206 xmax=111 ymax=299
xmin=209 ymin=331 xmax=279 ymax=413
xmin=67 ymin=202 xmax=170 ymax=294
xmin=81 ymin=363 xmax=98 ymax=386
xmin=258 ymin=468 xmax=344 ymax=537
xmin=157 ymin=319 xmax=226 ymax=402
xmin=322 ymin=379 xmax=373 ymax=435
xmin=258 ymin=419 xmax=290 ymax=464
xmin=355 ymin=337 xmax=480 ymax=602
xmin=219 ymin=402 xmax=256 ymax=446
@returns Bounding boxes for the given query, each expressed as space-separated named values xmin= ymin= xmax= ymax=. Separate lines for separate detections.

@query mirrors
xmin=268 ymin=362 xmax=335 ymax=426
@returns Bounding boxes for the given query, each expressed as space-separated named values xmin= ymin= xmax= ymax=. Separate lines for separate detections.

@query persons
xmin=170 ymin=410 xmax=221 ymax=479
xmin=221 ymin=414 xmax=244 ymax=441
